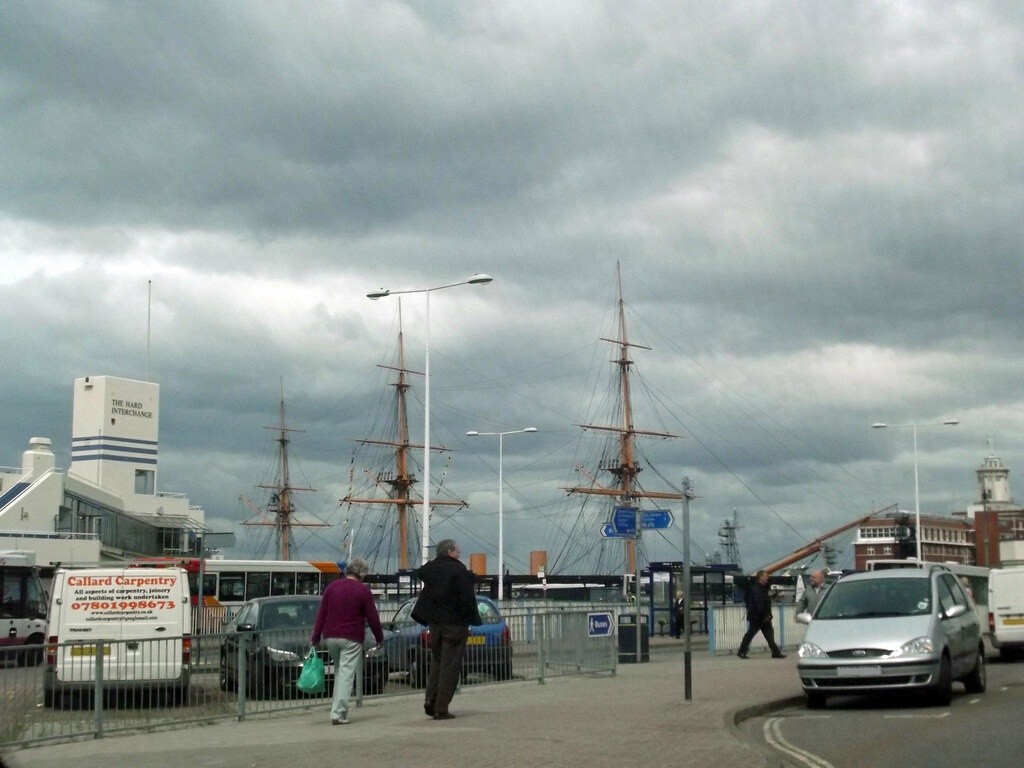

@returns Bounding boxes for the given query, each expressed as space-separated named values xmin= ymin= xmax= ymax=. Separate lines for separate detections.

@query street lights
xmin=872 ymin=421 xmax=960 ymax=560
xmin=468 ymin=428 xmax=539 ymax=601
xmin=364 ymin=275 xmax=495 ymax=567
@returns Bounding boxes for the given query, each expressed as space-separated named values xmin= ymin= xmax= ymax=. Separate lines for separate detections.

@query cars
xmin=796 ymin=563 xmax=987 ymax=705
xmin=218 ymin=594 xmax=389 ymax=696
xmin=380 ymin=597 xmax=514 ymax=688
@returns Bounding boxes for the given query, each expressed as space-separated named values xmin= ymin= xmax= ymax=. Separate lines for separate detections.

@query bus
xmin=131 ymin=560 xmax=347 ymax=635
xmin=866 ymin=559 xmax=989 ymax=635
xmin=0 ymin=551 xmax=49 ymax=664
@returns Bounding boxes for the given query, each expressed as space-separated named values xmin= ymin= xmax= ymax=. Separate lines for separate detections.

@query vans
xmin=986 ymin=566 xmax=1024 ymax=652
xmin=42 ymin=568 xmax=195 ymax=706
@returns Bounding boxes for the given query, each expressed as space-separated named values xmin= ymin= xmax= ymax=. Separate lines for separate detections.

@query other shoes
xmin=772 ymin=654 xmax=787 ymax=659
xmin=331 ymin=718 xmax=349 ymax=725
xmin=736 ymin=653 xmax=750 ymax=659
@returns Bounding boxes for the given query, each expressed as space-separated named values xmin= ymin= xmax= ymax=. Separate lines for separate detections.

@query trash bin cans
xmin=617 ymin=612 xmax=651 ymax=664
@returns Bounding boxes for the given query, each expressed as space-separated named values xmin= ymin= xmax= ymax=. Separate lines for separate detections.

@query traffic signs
xmin=600 ymin=506 xmax=675 ymax=539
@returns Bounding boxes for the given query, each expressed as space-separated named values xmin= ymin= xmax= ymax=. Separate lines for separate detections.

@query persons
xmin=409 ymin=538 xmax=483 ymax=721
xmin=674 ymin=591 xmax=685 ymax=639
xmin=310 ymin=556 xmax=384 ymax=725
xmin=737 ymin=570 xmax=788 ymax=659
xmin=796 ymin=570 xmax=830 ymax=617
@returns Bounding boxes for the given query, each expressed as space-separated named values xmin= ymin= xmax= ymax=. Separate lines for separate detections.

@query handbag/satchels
xmin=296 ymin=646 xmax=325 ymax=693
